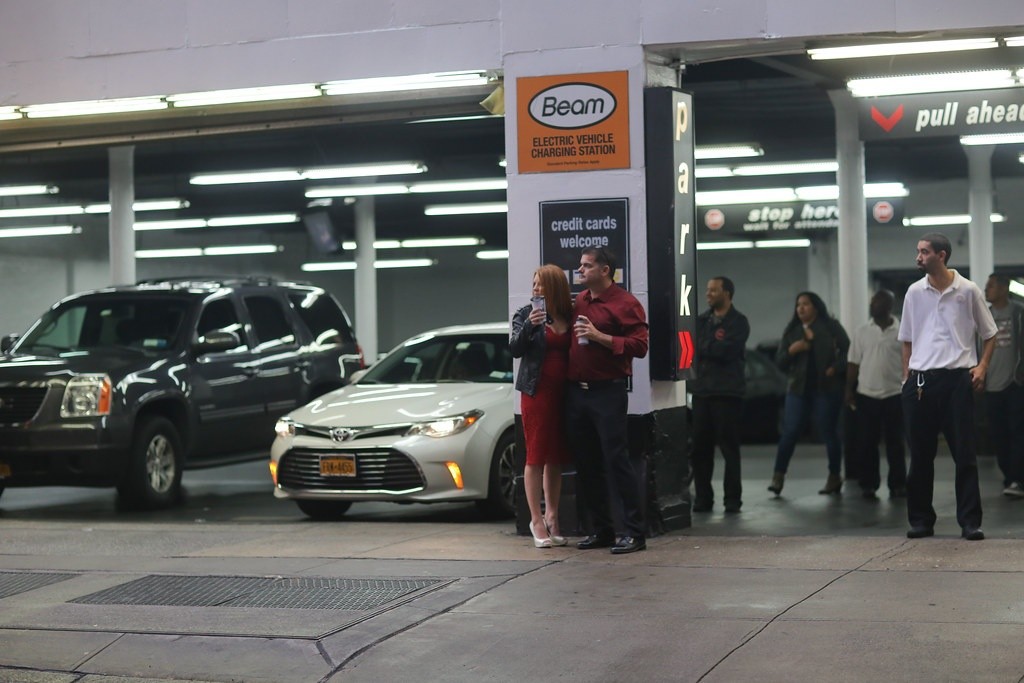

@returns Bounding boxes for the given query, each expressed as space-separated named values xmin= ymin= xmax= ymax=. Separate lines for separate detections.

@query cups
xmin=530 ymin=296 xmax=547 ymax=323
xmin=575 ymin=317 xmax=589 ymax=345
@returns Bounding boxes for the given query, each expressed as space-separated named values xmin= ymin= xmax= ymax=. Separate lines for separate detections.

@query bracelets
xmin=901 ymin=380 xmax=908 ymax=385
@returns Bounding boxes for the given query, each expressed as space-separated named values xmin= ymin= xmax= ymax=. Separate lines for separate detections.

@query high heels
xmin=543 ymin=518 xmax=568 ymax=545
xmin=529 ymin=521 xmax=552 ymax=548
xmin=819 ymin=477 xmax=843 ymax=493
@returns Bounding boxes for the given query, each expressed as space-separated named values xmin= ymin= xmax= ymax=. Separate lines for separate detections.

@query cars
xmin=269 ymin=323 xmax=517 ymax=521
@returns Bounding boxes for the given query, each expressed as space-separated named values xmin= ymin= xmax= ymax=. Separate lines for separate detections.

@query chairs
xmin=116 ymin=318 xmax=146 ymax=347
xmin=450 ymin=346 xmax=491 ymax=382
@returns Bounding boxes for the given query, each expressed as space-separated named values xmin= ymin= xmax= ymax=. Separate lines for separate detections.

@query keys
xmin=917 ymin=388 xmax=922 ymax=400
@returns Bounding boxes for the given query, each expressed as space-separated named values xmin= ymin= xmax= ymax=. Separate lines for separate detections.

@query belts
xmin=576 ymin=378 xmax=626 ymax=389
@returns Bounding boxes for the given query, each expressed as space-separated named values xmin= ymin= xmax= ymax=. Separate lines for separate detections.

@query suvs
xmin=0 ymin=276 xmax=367 ymax=509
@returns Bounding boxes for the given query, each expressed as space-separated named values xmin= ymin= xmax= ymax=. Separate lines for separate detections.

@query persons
xmin=897 ymin=233 xmax=999 ymax=541
xmin=570 ymin=244 xmax=649 ymax=553
xmin=977 ymin=272 xmax=1024 ymax=497
xmin=767 ymin=292 xmax=851 ymax=497
xmin=839 ymin=290 xmax=909 ymax=498
xmin=508 ymin=265 xmax=572 ymax=549
xmin=688 ymin=277 xmax=751 ymax=513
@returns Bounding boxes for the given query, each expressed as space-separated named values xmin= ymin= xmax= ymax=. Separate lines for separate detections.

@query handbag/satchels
xmin=828 ymin=320 xmax=847 ymax=382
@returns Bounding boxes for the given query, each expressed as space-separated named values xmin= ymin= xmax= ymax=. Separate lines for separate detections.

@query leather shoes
xmin=907 ymin=525 xmax=934 ymax=538
xmin=576 ymin=526 xmax=616 ymax=549
xmin=611 ymin=535 xmax=646 ymax=553
xmin=962 ymin=524 xmax=984 ymax=540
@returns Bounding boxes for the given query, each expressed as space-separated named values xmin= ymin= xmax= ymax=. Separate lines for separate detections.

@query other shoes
xmin=768 ymin=481 xmax=783 ymax=495
xmin=890 ymin=486 xmax=905 ymax=496
xmin=1003 ymin=481 xmax=1024 ymax=497
xmin=727 ymin=503 xmax=740 ymax=514
xmin=861 ymin=492 xmax=879 ymax=503
xmin=693 ymin=499 xmax=714 ymax=512
